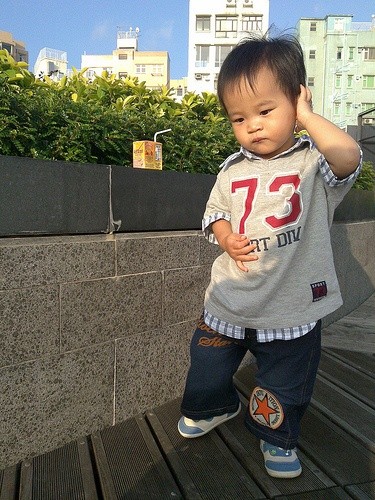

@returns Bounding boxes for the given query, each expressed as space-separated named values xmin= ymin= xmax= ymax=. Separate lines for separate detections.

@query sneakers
xmin=177 ymin=402 xmax=242 ymax=439
xmin=258 ymin=437 xmax=302 ymax=479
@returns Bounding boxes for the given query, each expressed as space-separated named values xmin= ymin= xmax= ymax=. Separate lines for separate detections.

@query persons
xmin=178 ymin=34 xmax=363 ymax=478
